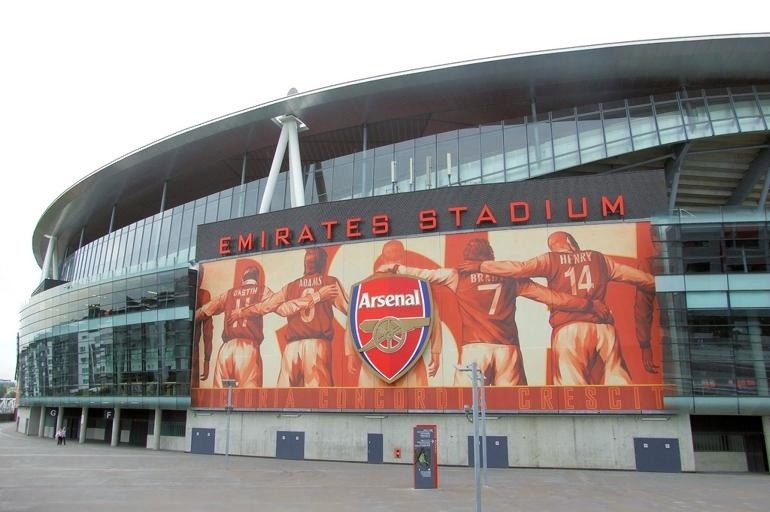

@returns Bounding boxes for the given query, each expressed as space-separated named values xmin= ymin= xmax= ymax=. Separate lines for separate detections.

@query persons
xmin=54 ymin=428 xmax=62 ymax=446
xmin=343 ymin=240 xmax=443 ymax=387
xmin=224 ymin=246 xmax=349 ymax=388
xmin=61 ymin=427 xmax=66 ymax=445
xmin=634 ymin=223 xmax=662 ymax=373
xmin=375 ymin=237 xmax=611 ymax=386
xmin=191 ymin=263 xmax=213 ymax=388
xmin=459 ymin=231 xmax=656 ymax=386
xmin=194 ymin=264 xmax=338 ymax=390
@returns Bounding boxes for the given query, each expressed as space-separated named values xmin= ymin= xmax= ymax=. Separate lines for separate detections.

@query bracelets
xmin=640 ymin=341 xmax=651 ymax=349
xmin=204 ymin=352 xmax=210 ymax=362
